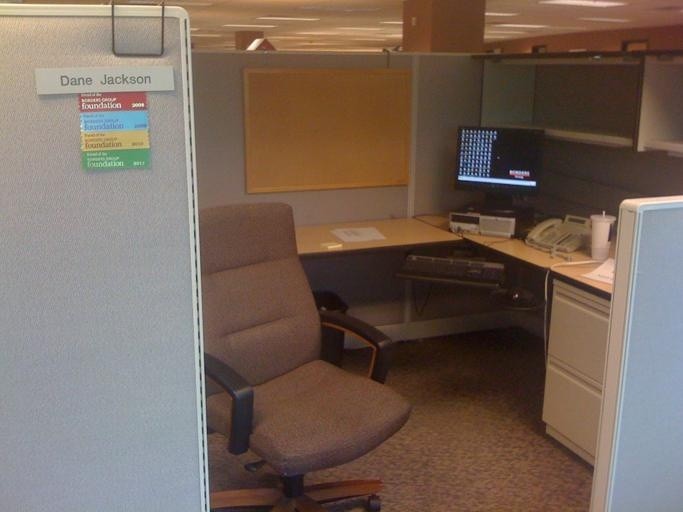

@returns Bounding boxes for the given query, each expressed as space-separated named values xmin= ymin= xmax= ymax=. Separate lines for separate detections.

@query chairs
xmin=195 ymin=201 xmax=411 ymax=510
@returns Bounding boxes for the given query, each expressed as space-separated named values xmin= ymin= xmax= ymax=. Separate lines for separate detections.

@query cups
xmin=590 ymin=214 xmax=616 ymax=260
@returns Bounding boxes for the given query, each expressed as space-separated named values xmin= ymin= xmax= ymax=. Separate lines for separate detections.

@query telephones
xmin=524 ymin=215 xmax=589 ymax=252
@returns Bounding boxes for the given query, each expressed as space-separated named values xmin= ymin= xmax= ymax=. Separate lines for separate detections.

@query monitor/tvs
xmin=456 ymin=127 xmax=545 ymax=214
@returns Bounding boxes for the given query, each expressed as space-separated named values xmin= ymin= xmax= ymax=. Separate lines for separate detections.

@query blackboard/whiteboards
xmin=243 ymin=67 xmax=409 ymax=194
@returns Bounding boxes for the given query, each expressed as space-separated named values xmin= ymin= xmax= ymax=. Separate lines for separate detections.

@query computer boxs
xmin=450 ymin=201 xmax=535 ymax=238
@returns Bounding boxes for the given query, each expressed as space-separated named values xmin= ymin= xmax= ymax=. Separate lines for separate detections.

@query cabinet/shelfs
xmin=541 ymin=276 xmax=614 ymax=466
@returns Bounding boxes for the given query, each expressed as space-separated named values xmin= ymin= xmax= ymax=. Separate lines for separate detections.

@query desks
xmin=416 ymin=215 xmax=614 ymax=302
xmin=294 ymin=217 xmax=464 ymax=257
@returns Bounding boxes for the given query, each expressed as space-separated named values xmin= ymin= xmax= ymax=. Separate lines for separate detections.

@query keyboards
xmin=399 ymin=253 xmax=505 ymax=283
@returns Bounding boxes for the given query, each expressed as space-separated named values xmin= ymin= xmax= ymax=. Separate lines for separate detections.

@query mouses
xmin=507 ymin=284 xmax=522 ymax=302
